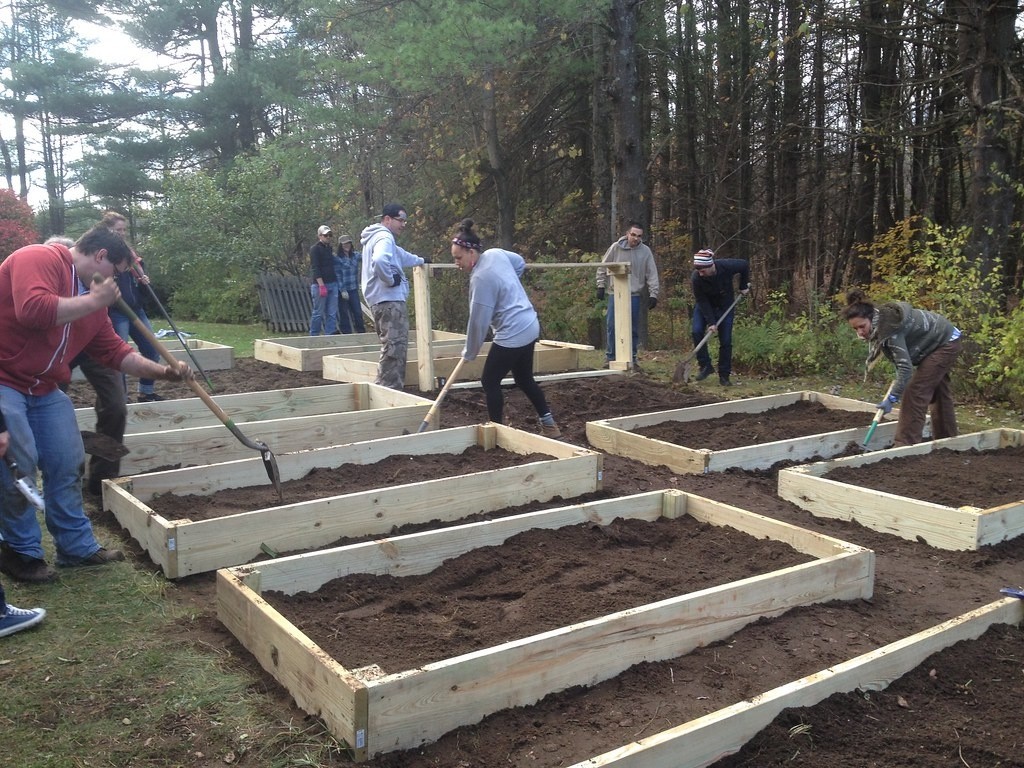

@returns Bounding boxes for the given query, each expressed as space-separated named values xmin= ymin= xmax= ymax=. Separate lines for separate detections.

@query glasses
xmin=321 ymin=234 xmax=332 ymax=237
xmin=113 ymin=264 xmax=121 ymax=282
xmin=393 ymin=217 xmax=408 ymax=225
xmin=629 ymin=231 xmax=642 ymax=239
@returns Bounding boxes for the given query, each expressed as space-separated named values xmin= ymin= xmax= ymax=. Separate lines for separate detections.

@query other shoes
xmin=633 ymin=363 xmax=643 ymax=372
xmin=602 ymin=359 xmax=614 ymax=368
xmin=695 ymin=365 xmax=715 ymax=381
xmin=136 ymin=392 xmax=171 ymax=402
xmin=540 ymin=423 xmax=562 ymax=439
xmin=720 ymin=377 xmax=733 ymax=387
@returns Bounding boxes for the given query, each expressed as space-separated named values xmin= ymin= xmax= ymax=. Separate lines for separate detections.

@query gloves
xmin=341 ymin=291 xmax=349 ymax=299
xmin=647 ymin=297 xmax=657 ymax=310
xmin=319 ymin=286 xmax=327 ymax=297
xmin=597 ymin=288 xmax=605 ymax=301
xmin=876 ymin=400 xmax=892 ymax=413
xmin=392 ymin=274 xmax=402 ymax=287
xmin=424 ymin=257 xmax=433 ymax=263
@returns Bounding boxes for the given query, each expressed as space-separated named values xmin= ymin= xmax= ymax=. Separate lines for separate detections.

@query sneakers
xmin=54 ymin=546 xmax=124 ymax=569
xmin=0 ymin=541 xmax=60 ymax=585
xmin=0 ymin=604 xmax=46 ymax=637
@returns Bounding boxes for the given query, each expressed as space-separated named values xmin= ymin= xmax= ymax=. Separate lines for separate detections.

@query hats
xmin=694 ymin=249 xmax=715 ymax=270
xmin=318 ymin=225 xmax=332 ymax=237
xmin=338 ymin=235 xmax=353 ymax=246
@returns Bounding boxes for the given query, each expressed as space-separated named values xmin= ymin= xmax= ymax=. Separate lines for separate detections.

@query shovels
xmin=673 ymin=289 xmax=750 ymax=383
xmin=133 ymin=264 xmax=224 ymax=396
xmin=93 ymin=272 xmax=282 ymax=504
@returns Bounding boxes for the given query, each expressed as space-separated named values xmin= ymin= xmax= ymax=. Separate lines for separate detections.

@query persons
xmin=844 ymin=301 xmax=962 ymax=447
xmin=596 ymin=225 xmax=659 ymax=373
xmin=0 ymin=228 xmax=196 ymax=637
xmin=334 ymin=235 xmax=365 ymax=334
xmin=105 ymin=213 xmax=165 ymax=403
xmin=309 ymin=226 xmax=338 ymax=336
xmin=691 ymin=250 xmax=749 ymax=386
xmin=361 ymin=206 xmax=433 ymax=390
xmin=452 ymin=220 xmax=561 ymax=439
